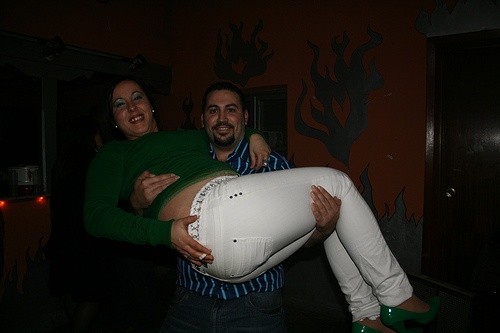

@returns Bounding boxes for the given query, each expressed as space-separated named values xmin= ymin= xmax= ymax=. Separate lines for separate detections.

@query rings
xmin=186 ymin=254 xmax=193 ymax=261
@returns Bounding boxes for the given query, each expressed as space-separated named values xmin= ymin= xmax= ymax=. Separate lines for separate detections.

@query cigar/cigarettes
xmin=198 ymin=248 xmax=212 ymax=261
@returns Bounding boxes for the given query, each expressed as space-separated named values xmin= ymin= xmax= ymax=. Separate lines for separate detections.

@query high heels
xmin=380 ymin=296 xmax=442 ymax=333
xmin=352 ymin=317 xmax=397 ymax=333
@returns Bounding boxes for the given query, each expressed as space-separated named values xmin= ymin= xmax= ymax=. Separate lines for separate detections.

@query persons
xmin=129 ymin=81 xmax=341 ymax=333
xmin=82 ymin=71 xmax=441 ymax=333
